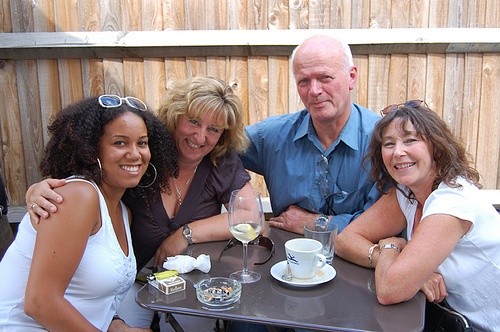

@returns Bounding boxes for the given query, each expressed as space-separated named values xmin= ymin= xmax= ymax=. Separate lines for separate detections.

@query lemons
xmin=234 ymin=223 xmax=256 ymax=240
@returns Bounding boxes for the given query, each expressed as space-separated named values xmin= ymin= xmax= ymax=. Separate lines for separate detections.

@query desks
xmin=135 ymin=220 xmax=426 ymax=332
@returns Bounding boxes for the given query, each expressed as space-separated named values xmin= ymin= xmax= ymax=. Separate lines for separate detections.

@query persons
xmin=334 ymin=99 xmax=500 ymax=332
xmin=239 ymin=35 xmax=390 ymax=240
xmin=0 ymin=95 xmax=178 ymax=332
xmin=25 ymin=76 xmax=260 ymax=332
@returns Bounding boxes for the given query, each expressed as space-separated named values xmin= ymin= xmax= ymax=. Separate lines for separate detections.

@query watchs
xmin=183 ymin=223 xmax=193 ymax=245
xmin=314 ymin=215 xmax=329 ymax=231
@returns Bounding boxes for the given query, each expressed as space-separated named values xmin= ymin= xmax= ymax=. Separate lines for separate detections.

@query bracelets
xmin=113 ymin=316 xmax=125 ymax=323
xmin=368 ymin=244 xmax=379 ymax=267
xmin=380 ymin=243 xmax=401 ymax=253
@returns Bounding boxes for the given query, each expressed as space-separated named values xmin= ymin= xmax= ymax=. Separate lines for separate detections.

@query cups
xmin=285 ymin=238 xmax=326 ymax=279
xmin=304 ymin=221 xmax=338 ymax=265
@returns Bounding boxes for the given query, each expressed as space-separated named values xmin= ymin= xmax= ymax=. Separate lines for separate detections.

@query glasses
xmin=97 ymin=94 xmax=148 ymax=112
xmin=380 ymin=100 xmax=431 ymax=118
xmin=317 ymin=154 xmax=329 ymax=196
xmin=219 ymin=236 xmax=276 ymax=266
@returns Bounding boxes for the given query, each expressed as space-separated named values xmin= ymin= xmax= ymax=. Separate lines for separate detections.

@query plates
xmin=270 ymin=259 xmax=336 ymax=288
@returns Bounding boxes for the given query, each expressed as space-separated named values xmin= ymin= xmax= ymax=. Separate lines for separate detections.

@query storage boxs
xmin=147 ymin=277 xmax=186 ymax=294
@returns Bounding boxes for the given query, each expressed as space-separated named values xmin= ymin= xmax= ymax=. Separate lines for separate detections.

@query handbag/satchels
xmin=423 ymin=297 xmax=474 ymax=332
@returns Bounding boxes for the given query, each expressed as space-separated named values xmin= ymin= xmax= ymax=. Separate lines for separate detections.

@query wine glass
xmin=228 ymin=189 xmax=264 ymax=283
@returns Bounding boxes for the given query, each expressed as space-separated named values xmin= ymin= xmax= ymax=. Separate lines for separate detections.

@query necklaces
xmin=171 ymin=165 xmax=198 ymax=205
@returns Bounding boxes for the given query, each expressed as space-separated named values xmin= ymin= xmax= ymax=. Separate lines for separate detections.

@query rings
xmin=31 ymin=203 xmax=37 ymax=210
